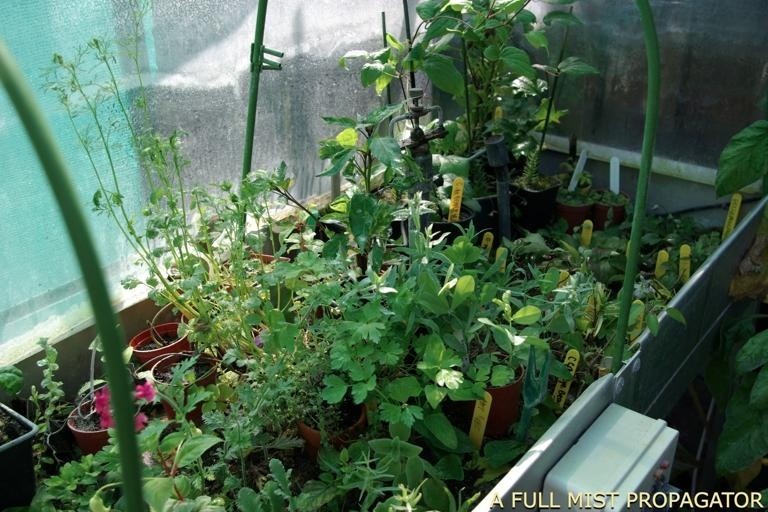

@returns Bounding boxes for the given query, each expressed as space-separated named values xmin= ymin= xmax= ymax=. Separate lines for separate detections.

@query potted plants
xmin=0 ymin=0 xmax=726 ymax=512
